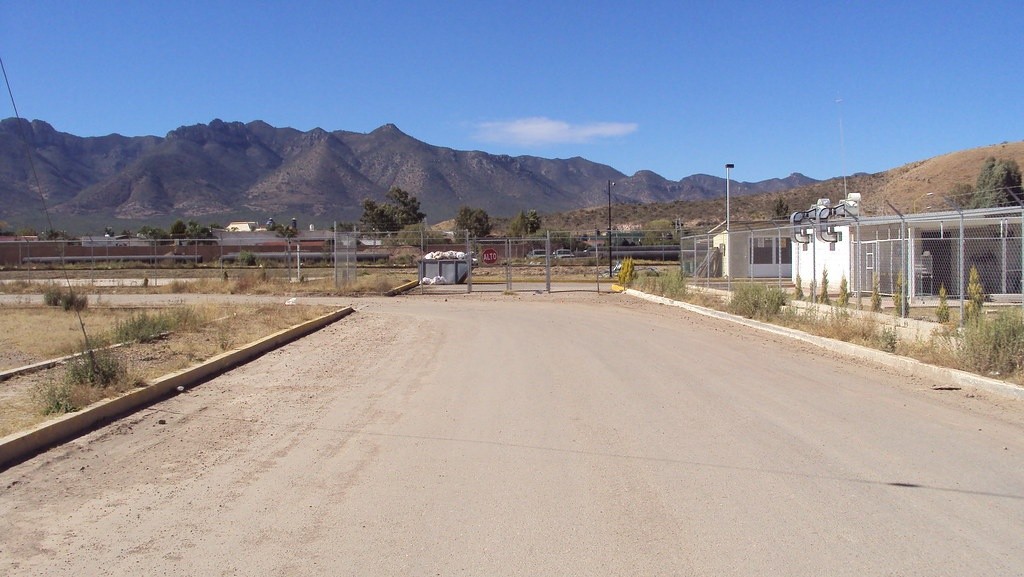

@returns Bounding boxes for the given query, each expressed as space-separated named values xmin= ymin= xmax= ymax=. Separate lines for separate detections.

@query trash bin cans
xmin=417 ymin=251 xmax=478 ymax=285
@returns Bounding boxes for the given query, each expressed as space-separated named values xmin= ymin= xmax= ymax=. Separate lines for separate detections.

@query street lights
xmin=725 ymin=164 xmax=735 ymax=278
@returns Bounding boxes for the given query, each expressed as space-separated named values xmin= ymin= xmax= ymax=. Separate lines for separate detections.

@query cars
xmin=526 ymin=249 xmax=546 ymax=261
xmin=555 ymin=249 xmax=574 ymax=260
